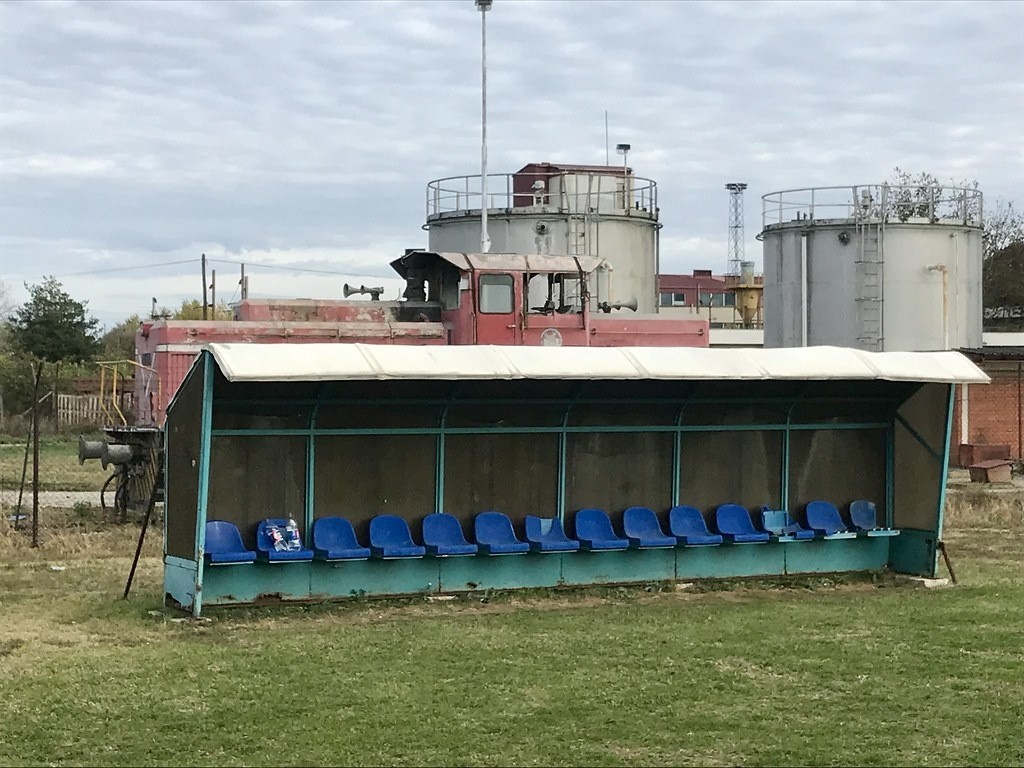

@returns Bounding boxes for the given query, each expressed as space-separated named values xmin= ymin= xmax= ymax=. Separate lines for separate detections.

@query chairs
xmin=204 ymin=496 xmax=902 ymax=564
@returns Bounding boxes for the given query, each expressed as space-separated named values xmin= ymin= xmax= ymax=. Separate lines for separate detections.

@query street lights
xmin=474 ymin=0 xmax=494 ymax=256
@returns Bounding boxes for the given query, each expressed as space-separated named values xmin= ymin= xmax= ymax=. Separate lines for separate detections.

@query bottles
xmin=286 ymin=513 xmax=301 ymax=552
xmin=264 ymin=519 xmax=288 ymax=552
